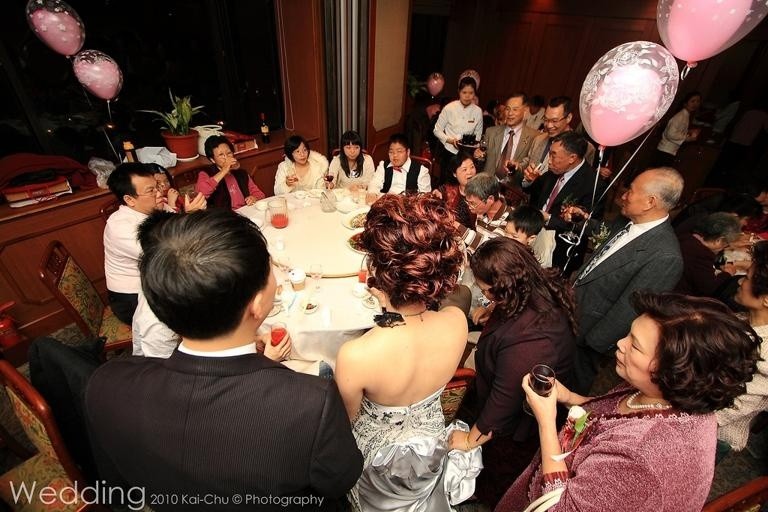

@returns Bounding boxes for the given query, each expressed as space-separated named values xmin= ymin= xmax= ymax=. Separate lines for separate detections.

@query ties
xmin=544 ymin=178 xmax=563 ymax=213
xmin=540 ymin=137 xmax=555 ymax=164
xmin=495 ymin=131 xmax=515 ymax=180
xmin=576 ymin=223 xmax=631 ymax=280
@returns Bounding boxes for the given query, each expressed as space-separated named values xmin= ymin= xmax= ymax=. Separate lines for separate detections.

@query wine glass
xmin=363 ymin=269 xmax=382 ymax=309
xmin=565 ymin=204 xmax=586 ymax=239
xmin=524 ymin=363 xmax=555 ymax=418
xmin=310 ymin=263 xmax=324 ymax=292
xmin=324 ymin=167 xmax=334 ymax=193
xmin=478 ymin=141 xmax=487 ymax=160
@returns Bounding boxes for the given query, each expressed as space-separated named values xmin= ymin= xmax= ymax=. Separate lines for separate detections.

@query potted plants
xmin=137 ymin=86 xmax=205 ymax=158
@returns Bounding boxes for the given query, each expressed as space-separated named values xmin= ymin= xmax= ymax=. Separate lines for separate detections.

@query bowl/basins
xmin=293 ymin=191 xmax=308 ymax=199
xmin=299 ymin=300 xmax=319 ymax=314
xmin=253 ymin=200 xmax=268 ymax=211
xmin=352 ymin=283 xmax=368 ymax=297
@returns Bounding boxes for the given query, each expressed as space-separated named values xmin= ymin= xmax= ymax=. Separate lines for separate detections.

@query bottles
xmin=120 ymin=134 xmax=138 ymax=163
xmin=260 ymin=113 xmax=271 ymax=144
xmin=714 ymin=250 xmax=725 ymax=270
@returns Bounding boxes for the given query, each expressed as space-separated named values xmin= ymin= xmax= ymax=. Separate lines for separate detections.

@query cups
xmin=359 ymin=270 xmax=367 ymax=283
xmin=351 ymin=182 xmax=378 ymax=206
xmin=267 ymin=197 xmax=288 ymax=229
xmin=288 ymin=269 xmax=307 ymax=292
xmin=320 ymin=190 xmax=338 ymax=212
xmin=461 ymin=130 xmax=478 ymax=145
xmin=271 ymin=322 xmax=286 ymax=347
xmin=507 ymin=160 xmax=516 ymax=177
xmin=523 ymin=161 xmax=545 ymax=183
xmin=229 ymin=160 xmax=241 ymax=171
xmin=287 ymin=169 xmax=299 ymax=185
xmin=185 ymin=184 xmax=199 ymax=203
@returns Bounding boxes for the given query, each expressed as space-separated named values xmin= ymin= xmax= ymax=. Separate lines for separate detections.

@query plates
xmin=345 ymin=228 xmax=371 ymax=255
xmin=249 ymin=217 xmax=264 ymax=227
xmin=308 ymin=189 xmax=323 ymax=198
xmin=268 ymin=288 xmax=296 ymax=317
xmin=342 ymin=206 xmax=371 ymax=230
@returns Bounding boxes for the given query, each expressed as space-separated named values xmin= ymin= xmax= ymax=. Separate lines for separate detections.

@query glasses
xmin=136 ymin=183 xmax=163 ymax=197
xmin=464 ymin=198 xmax=484 ymax=209
xmin=540 ymin=115 xmax=565 ymax=125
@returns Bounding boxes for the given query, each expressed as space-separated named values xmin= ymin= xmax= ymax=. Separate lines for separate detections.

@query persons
xmin=101 ymin=159 xmax=207 ymax=326
xmin=332 ymin=189 xmax=470 ymax=512
xmin=326 ymin=129 xmax=375 ymax=195
xmin=79 ymin=206 xmax=364 ymax=512
xmin=422 ymin=66 xmax=766 ymax=512
xmin=273 ymin=134 xmax=331 ymax=198
xmin=369 ymin=134 xmax=435 ymax=198
xmin=144 ymin=164 xmax=194 ymax=215
xmin=196 ymin=134 xmax=265 ymax=214
xmin=574 ymin=39 xmax=681 ymax=154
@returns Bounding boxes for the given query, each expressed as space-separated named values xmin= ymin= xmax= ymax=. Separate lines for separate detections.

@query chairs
xmin=440 ymin=367 xmax=476 ymax=428
xmin=702 ymin=475 xmax=768 ymax=512
xmin=499 ymin=182 xmax=528 ymax=207
xmin=38 ymin=239 xmax=132 ymax=351
xmin=410 ymin=156 xmax=433 ymax=175
xmin=0 ymin=361 xmax=111 ymax=512
xmin=331 ymin=148 xmax=368 ymax=157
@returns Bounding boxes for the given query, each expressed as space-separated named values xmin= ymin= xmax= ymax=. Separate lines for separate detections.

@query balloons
xmin=23 ymin=0 xmax=89 ymax=63
xmin=653 ymin=0 xmax=768 ymax=72
xmin=73 ymin=48 xmax=125 ymax=103
